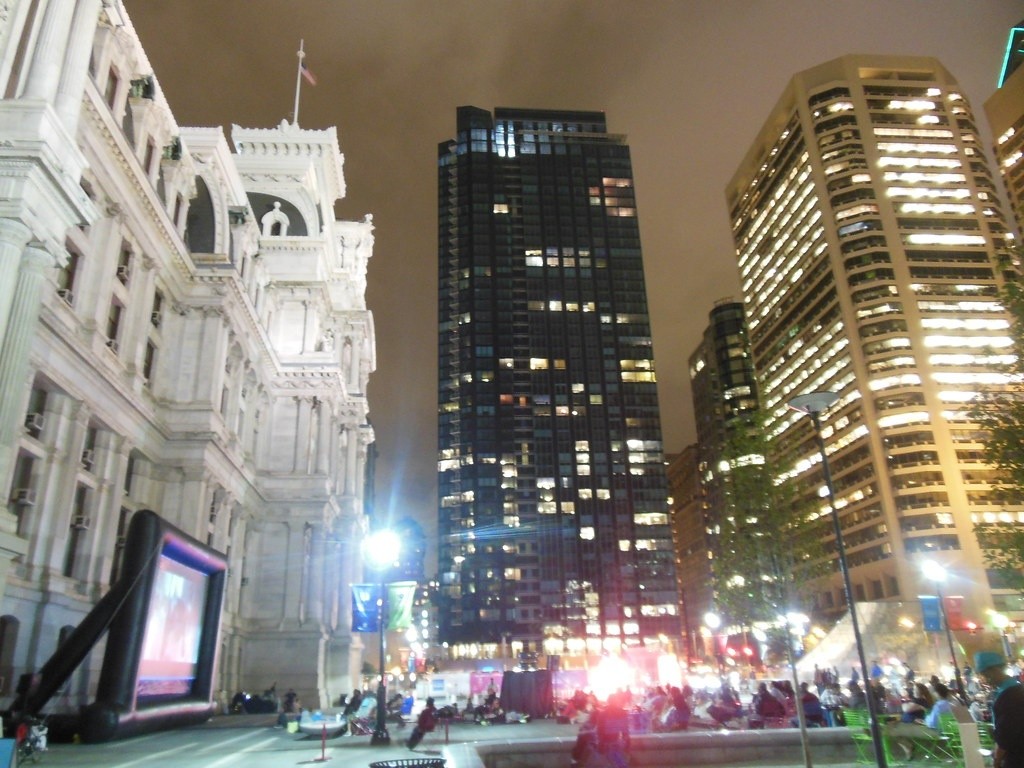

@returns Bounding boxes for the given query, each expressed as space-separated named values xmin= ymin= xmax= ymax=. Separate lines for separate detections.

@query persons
xmin=462 ymin=698 xmax=474 ymax=720
xmin=473 ymin=689 xmax=506 ymax=725
xmin=271 ymin=682 xmax=277 ymax=692
xmin=386 ymin=694 xmax=409 ymax=727
xmin=341 ymin=689 xmax=377 ymax=736
xmin=453 ymin=703 xmax=461 ymax=719
xmin=405 ymin=697 xmax=439 ymax=750
xmin=558 ymin=688 xmax=599 ymax=717
xmin=282 ymin=689 xmax=297 ymax=711
xmin=294 ymin=698 xmax=303 ymax=715
xmin=616 ymin=678 xmax=743 ymax=731
xmin=579 ymin=694 xmax=632 ymax=768
xmin=750 ymin=650 xmax=1024 ymax=768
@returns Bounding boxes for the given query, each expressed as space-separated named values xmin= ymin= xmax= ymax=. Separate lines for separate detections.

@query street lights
xmin=789 ymin=389 xmax=890 ymax=768
xmin=366 ymin=526 xmax=400 ymax=746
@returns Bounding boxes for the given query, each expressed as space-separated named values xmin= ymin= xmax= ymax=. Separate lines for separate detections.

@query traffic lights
xmin=944 ymin=594 xmax=967 ymax=632
xmin=915 ymin=594 xmax=943 ymax=632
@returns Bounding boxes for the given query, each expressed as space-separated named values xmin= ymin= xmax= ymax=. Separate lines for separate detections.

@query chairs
xmin=843 ymin=710 xmax=996 ymax=768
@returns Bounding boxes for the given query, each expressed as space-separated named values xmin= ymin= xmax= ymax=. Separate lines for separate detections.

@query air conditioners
xmin=151 ymin=311 xmax=162 ymax=325
xmin=15 ymin=487 xmax=36 ymax=507
xmin=107 ymin=339 xmax=120 ymax=354
xmin=25 ymin=412 xmax=47 ymax=431
xmin=117 ymin=535 xmax=127 ymax=547
xmin=82 ymin=449 xmax=97 ymax=466
xmin=72 ymin=515 xmax=90 ymax=531
xmin=118 ymin=266 xmax=131 ymax=281
xmin=57 ymin=289 xmax=76 ymax=305
